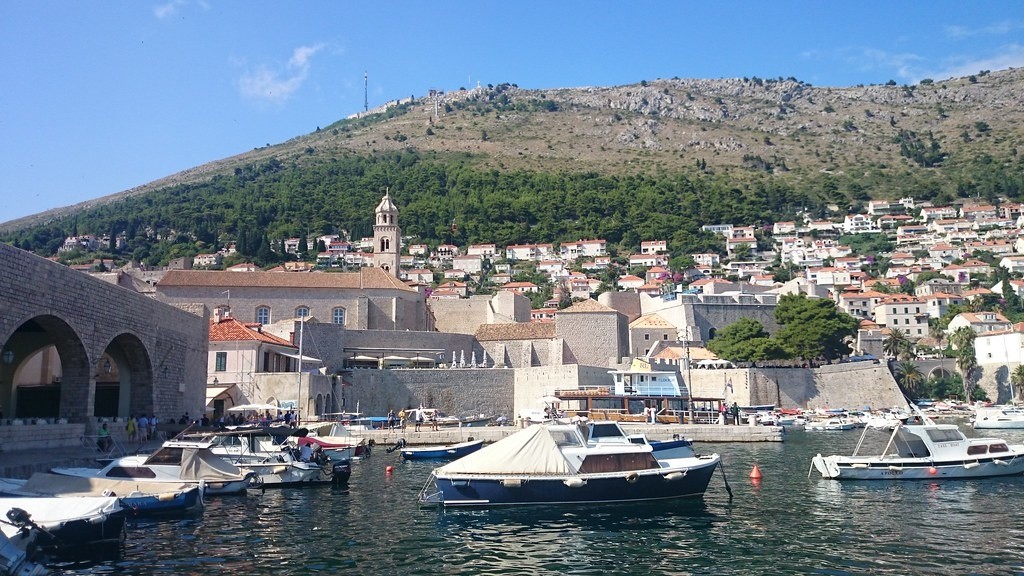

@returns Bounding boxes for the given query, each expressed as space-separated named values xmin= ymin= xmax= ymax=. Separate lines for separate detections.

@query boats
xmin=417 ymin=419 xmax=723 ymax=507
xmin=554 ymin=348 xmax=748 ymax=426
xmin=0 ymin=420 xmax=374 ymax=550
xmin=406 ymin=409 xmax=496 ymax=426
xmin=807 ymin=395 xmax=1024 ymax=484
xmin=749 ymin=400 xmax=1022 ymax=431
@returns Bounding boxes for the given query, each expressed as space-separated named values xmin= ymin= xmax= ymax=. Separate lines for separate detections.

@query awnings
xmin=275 ymin=351 xmax=322 ymax=363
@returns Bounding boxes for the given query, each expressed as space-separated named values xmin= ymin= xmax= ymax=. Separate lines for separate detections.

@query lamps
xmin=94 ymin=358 xmax=112 ymax=373
xmin=207 ymin=376 xmax=219 ymax=386
xmin=161 ymin=365 xmax=170 ymax=379
xmin=0 ymin=344 xmax=15 ymax=364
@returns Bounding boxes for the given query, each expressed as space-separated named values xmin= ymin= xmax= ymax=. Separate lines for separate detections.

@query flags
xmin=727 ymin=378 xmax=733 ymax=393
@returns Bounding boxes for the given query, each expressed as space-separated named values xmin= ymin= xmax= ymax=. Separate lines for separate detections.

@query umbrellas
xmin=227 ymin=403 xmax=283 ymax=418
xmin=839 ymin=353 xmax=877 ymax=363
xmin=541 ymin=395 xmax=563 ymax=404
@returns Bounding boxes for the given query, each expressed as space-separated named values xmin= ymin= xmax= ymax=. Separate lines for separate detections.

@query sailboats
xmin=401 ymin=439 xmax=485 ymax=459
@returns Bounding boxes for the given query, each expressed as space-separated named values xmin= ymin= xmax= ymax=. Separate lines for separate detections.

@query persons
xmin=398 ymin=408 xmax=407 ymax=433
xmin=127 ymin=413 xmax=159 ymax=445
xmin=431 ymin=410 xmax=439 ymax=431
xmin=543 ymin=408 xmax=567 ymax=418
xmin=388 ymin=410 xmax=396 ymax=433
xmin=731 ymin=402 xmax=741 ymax=425
xmin=722 ymin=403 xmax=728 ymax=425
xmin=97 ymin=423 xmax=113 ymax=452
xmin=644 ymin=406 xmax=656 ymax=425
xmin=179 ymin=410 xmax=301 ymax=429
xmin=415 ymin=407 xmax=423 ymax=432
